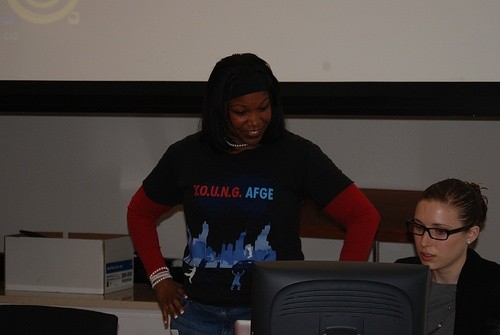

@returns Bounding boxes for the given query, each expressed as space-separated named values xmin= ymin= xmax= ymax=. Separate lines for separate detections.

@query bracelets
xmin=150 ymin=267 xmax=173 ymax=288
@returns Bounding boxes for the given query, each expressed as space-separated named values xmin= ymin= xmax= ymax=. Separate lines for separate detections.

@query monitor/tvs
xmin=250 ymin=260 xmax=431 ymax=335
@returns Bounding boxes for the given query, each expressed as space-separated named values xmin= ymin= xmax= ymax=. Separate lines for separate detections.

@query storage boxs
xmin=3 ymin=230 xmax=136 ymax=296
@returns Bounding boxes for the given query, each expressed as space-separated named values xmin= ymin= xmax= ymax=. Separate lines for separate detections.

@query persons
xmin=393 ymin=179 xmax=500 ymax=335
xmin=125 ymin=54 xmax=382 ymax=334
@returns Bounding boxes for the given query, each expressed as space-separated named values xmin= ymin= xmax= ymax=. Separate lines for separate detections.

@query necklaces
xmin=224 ymin=136 xmax=248 ymax=148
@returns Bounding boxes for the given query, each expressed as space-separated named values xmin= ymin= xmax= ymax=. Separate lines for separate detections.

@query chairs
xmin=1 ymin=304 xmax=120 ymax=335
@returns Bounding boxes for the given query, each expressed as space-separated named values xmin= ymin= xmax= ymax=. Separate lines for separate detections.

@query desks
xmin=2 ymin=283 xmax=171 ymax=334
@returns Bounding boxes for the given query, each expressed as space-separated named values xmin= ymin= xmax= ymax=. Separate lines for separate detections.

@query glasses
xmin=407 ymin=218 xmax=472 ymax=240
